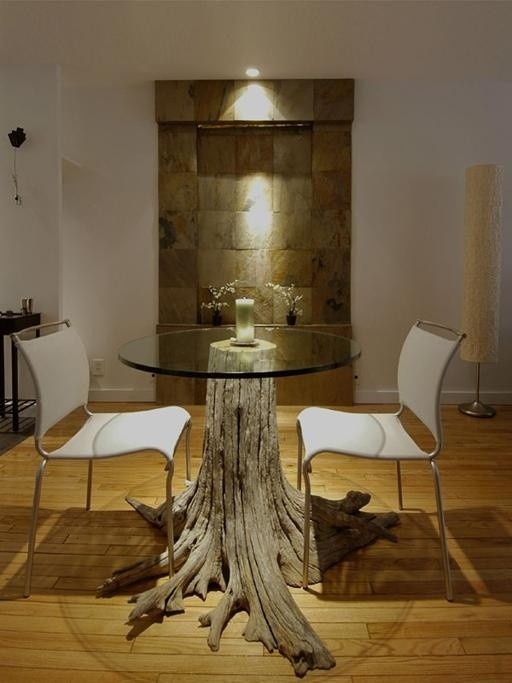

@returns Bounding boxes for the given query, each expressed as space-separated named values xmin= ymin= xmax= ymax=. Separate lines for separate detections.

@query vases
xmin=212 ymin=311 xmax=222 ymax=326
xmin=287 ymin=311 xmax=296 ymax=325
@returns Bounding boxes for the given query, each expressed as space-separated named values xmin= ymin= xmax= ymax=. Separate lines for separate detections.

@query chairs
xmin=10 ymin=318 xmax=192 ymax=598
xmin=297 ymin=319 xmax=467 ymax=602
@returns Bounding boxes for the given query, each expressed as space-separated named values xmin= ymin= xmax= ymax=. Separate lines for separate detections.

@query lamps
xmin=459 ymin=164 xmax=503 ymax=418
xmin=8 ymin=127 xmax=27 ymax=200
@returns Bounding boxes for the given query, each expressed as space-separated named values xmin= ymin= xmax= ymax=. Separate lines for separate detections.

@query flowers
xmin=201 ymin=280 xmax=238 ymax=312
xmin=265 ymin=282 xmax=304 ymax=316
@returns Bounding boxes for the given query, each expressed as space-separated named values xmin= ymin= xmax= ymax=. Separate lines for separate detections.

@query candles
xmin=236 ymin=296 xmax=255 ymax=343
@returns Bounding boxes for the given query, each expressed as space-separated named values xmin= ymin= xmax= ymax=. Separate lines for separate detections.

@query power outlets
xmin=9 ymin=191 xmax=22 ymax=206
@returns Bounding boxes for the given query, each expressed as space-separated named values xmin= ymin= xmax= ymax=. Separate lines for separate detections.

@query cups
xmin=21 ymin=297 xmax=32 ymax=314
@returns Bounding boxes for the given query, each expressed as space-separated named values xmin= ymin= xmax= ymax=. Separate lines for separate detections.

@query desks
xmin=1 ymin=313 xmax=42 ymax=432
xmin=95 ymin=325 xmax=402 ymax=677
